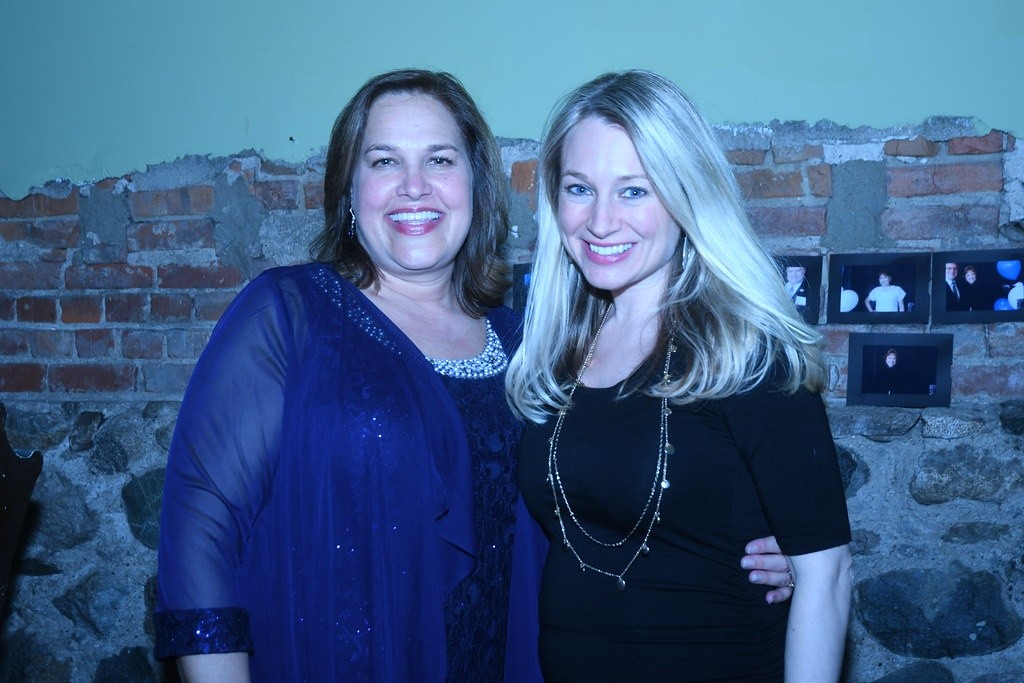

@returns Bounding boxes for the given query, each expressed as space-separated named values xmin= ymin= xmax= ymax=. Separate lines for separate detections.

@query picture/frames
xmin=932 ymin=248 xmax=1024 ymax=324
xmin=846 ymin=332 xmax=954 ymax=407
xmin=827 ymin=252 xmax=931 ymax=324
xmin=774 ymin=256 xmax=823 ymax=324
xmin=513 ymin=263 xmax=532 ymax=316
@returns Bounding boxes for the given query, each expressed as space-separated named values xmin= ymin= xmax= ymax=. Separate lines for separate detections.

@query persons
xmin=508 ymin=70 xmax=859 ymax=683
xmin=876 ymin=347 xmax=910 ymax=396
xmin=863 ymin=269 xmax=907 ymax=313
xmin=148 ymin=66 xmax=793 ymax=683
xmin=945 ymin=262 xmax=975 ymax=312
xmin=782 ymin=266 xmax=821 ymax=323
xmin=961 ymin=264 xmax=989 ymax=313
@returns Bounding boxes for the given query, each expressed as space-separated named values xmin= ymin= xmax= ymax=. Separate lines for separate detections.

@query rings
xmin=785 ymin=570 xmax=795 ymax=590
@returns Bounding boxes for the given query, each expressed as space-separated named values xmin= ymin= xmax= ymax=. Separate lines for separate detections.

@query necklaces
xmin=543 ymin=294 xmax=683 ymax=592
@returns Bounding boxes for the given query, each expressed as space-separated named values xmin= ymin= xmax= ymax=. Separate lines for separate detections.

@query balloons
xmin=1008 ymin=285 xmax=1024 ymax=310
xmin=993 ymin=299 xmax=1015 ymax=310
xmin=839 ymin=290 xmax=859 ymax=312
xmin=996 ymin=260 xmax=1022 ymax=281
